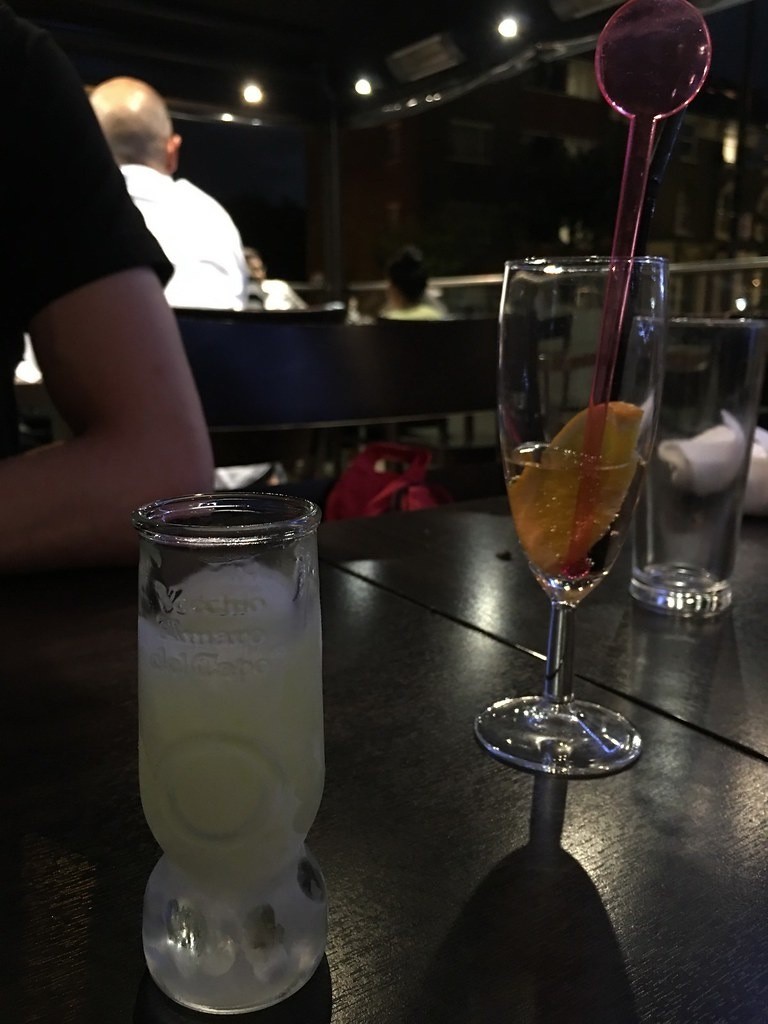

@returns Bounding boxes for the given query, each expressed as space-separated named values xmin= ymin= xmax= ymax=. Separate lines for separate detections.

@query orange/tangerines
xmin=507 ymin=400 xmax=644 ymax=574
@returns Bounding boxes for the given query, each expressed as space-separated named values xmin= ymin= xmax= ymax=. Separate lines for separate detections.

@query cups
xmin=130 ymin=490 xmax=330 ymax=1015
xmin=623 ymin=315 xmax=767 ymax=619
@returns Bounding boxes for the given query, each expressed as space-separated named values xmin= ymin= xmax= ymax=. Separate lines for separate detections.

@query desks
xmin=1 ymin=491 xmax=766 ymax=1023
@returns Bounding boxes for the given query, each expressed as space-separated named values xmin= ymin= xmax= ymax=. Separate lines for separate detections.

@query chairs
xmin=144 ymin=303 xmax=570 ymax=481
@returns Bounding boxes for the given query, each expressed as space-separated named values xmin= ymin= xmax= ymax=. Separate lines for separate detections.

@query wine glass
xmin=473 ymin=254 xmax=668 ymax=779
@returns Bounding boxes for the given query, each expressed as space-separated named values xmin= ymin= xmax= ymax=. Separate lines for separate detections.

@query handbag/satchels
xmin=326 ymin=439 xmax=454 ymax=520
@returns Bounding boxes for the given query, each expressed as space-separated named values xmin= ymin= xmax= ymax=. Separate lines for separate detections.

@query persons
xmin=0 ymin=5 xmax=215 ymax=571
xmin=242 ymin=247 xmax=305 ymax=314
xmin=16 ymin=78 xmax=277 ymax=489
xmin=378 ymin=248 xmax=445 ymax=321
